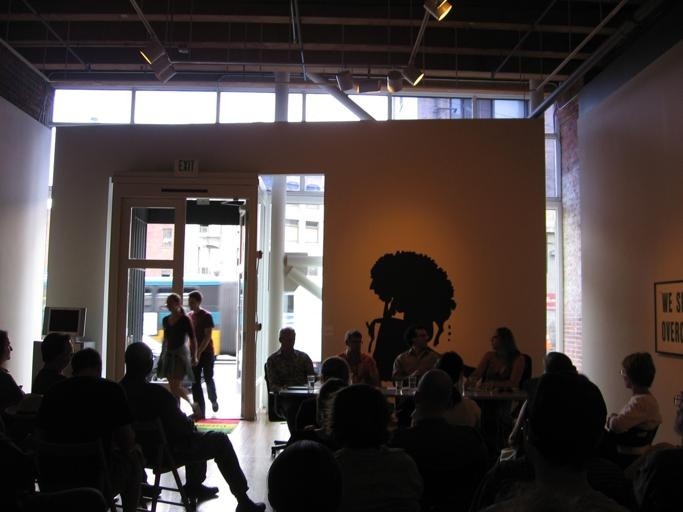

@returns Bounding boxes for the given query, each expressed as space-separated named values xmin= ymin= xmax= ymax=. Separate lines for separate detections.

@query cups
xmin=306 ymin=374 xmax=316 ymax=392
xmin=394 ymin=375 xmax=417 ymax=393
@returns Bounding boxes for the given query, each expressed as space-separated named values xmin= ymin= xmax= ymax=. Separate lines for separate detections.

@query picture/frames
xmin=654 ymin=280 xmax=683 ymax=357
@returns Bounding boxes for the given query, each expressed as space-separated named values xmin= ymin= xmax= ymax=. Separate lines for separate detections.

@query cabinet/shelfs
xmin=31 ymin=340 xmax=94 ymax=397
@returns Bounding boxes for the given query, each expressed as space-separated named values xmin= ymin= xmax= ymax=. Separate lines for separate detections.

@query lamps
xmin=138 ymin=29 xmax=177 ymax=86
xmin=402 ymin=0 xmax=460 ymax=86
xmin=332 ymin=61 xmax=403 ymax=95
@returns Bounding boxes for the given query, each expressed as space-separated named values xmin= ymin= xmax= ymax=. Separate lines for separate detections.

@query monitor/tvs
xmin=42 ymin=306 xmax=87 ymax=337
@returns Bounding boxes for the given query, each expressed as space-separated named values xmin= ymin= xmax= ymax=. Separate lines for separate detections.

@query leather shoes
xmin=234 ymin=499 xmax=266 ymax=511
xmin=180 ymin=482 xmax=219 ymax=501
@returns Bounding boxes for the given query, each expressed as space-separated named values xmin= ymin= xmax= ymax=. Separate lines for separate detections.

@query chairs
xmin=263 ymin=361 xmax=311 ymax=457
xmin=517 ymin=351 xmax=533 ymax=392
xmin=116 ymin=406 xmax=208 ymax=511
xmin=23 ymin=486 xmax=108 ymax=511
xmin=606 ymin=421 xmax=659 ymax=456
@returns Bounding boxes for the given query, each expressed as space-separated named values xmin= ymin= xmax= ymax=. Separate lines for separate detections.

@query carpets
xmin=193 ymin=417 xmax=240 ymax=436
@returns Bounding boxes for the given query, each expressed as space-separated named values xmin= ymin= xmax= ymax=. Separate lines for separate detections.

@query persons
xmin=30 ymin=333 xmax=162 ymax=500
xmin=187 ymin=288 xmax=219 ymax=423
xmin=263 ymin=326 xmax=317 ymax=435
xmin=0 ymin=437 xmax=106 ymax=512
xmin=0 ymin=331 xmax=25 ymax=410
xmin=156 ymin=293 xmax=202 ymax=423
xmin=293 ymin=322 xmax=683 ymax=512
xmin=266 ymin=439 xmax=343 ymax=511
xmin=117 ymin=341 xmax=265 ymax=512
xmin=33 ymin=347 xmax=150 ymax=510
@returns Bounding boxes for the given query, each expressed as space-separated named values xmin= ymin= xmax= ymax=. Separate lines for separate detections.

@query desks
xmin=279 ymin=377 xmax=542 ymax=430
